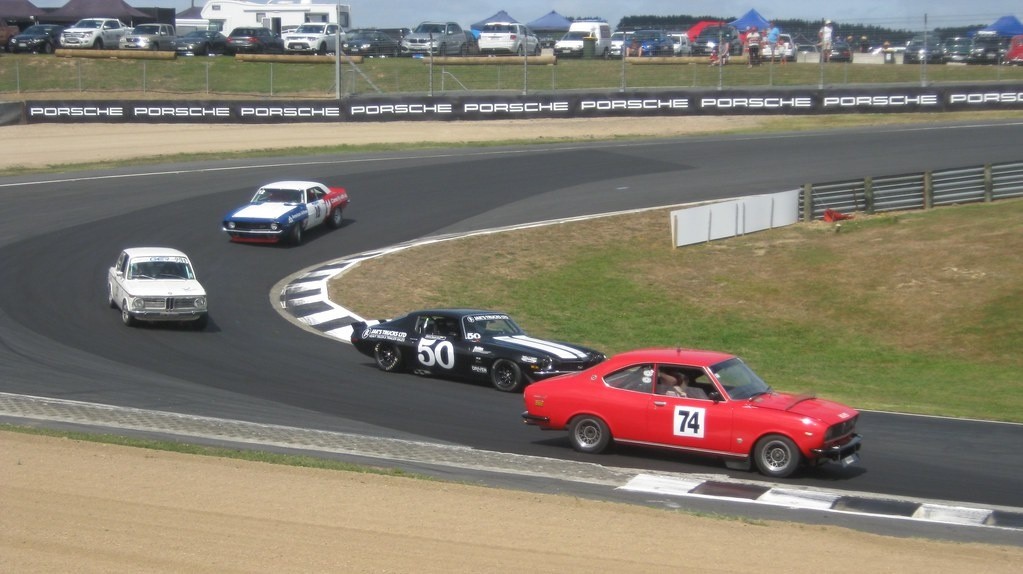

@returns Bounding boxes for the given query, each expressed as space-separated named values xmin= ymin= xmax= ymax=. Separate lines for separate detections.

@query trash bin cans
xmin=582 ymin=37 xmax=597 ymax=60
xmin=884 ymin=50 xmax=897 ymax=64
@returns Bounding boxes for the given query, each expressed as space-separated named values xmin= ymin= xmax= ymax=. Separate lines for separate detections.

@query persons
xmin=710 ymin=22 xmax=788 ymax=69
xmin=437 ymin=319 xmax=460 ymax=337
xmin=133 ymin=262 xmax=152 ymax=279
xmin=665 ymin=372 xmax=693 ymax=400
xmin=818 ymin=20 xmax=872 ymax=62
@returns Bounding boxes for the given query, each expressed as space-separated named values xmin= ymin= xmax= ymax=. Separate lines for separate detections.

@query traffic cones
xmin=823 ymin=208 xmax=854 ymax=222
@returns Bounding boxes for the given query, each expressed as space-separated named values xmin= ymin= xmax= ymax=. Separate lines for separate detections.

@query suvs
xmin=477 ymin=21 xmax=542 ymax=56
xmin=401 ymin=20 xmax=468 ymax=57
xmin=283 ymin=22 xmax=347 ymax=54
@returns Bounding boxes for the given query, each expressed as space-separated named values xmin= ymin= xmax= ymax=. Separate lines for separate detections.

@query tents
xmin=968 ymin=15 xmax=1023 ymax=37
xmin=0 ymin=0 xmax=153 ymax=25
xmin=727 ymin=9 xmax=771 ymax=33
xmin=470 ymin=9 xmax=573 ymax=42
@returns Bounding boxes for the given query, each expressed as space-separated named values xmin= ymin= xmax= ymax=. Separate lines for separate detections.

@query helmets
xmin=669 ymin=368 xmax=692 ymax=382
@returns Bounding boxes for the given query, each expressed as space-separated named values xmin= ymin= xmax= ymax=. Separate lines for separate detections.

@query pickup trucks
xmin=59 ymin=17 xmax=135 ymax=50
xmin=119 ymin=22 xmax=184 ymax=51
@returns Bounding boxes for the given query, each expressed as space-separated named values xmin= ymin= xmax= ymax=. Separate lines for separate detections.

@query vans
xmin=552 ymin=20 xmax=611 ymax=61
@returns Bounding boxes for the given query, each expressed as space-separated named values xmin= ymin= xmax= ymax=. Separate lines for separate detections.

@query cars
xmin=349 ymin=308 xmax=608 ymax=394
xmin=821 ymin=39 xmax=853 ymax=62
xmin=8 ymin=23 xmax=69 ymax=53
xmin=342 ymin=29 xmax=401 ymax=57
xmin=220 ymin=179 xmax=349 ymax=244
xmin=903 ymin=29 xmax=1023 ymax=67
xmin=169 ymin=30 xmax=228 ymax=56
xmin=520 ymin=345 xmax=865 ymax=478
xmin=226 ymin=27 xmax=283 ymax=55
xmin=107 ymin=245 xmax=208 ymax=326
xmin=608 ymin=23 xmax=744 ymax=57
xmin=758 ymin=33 xmax=799 ymax=62
